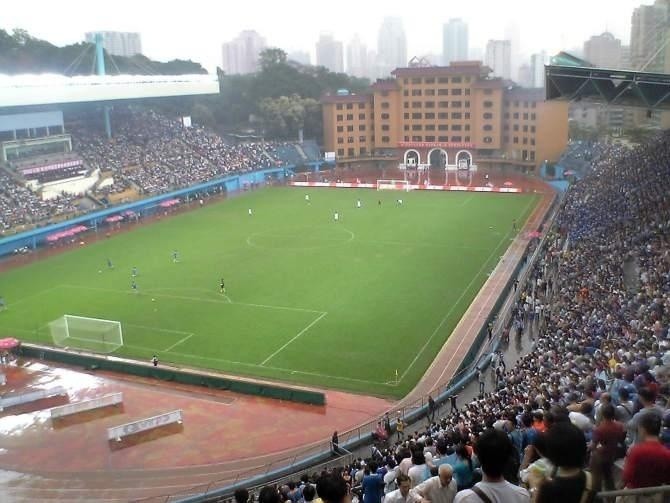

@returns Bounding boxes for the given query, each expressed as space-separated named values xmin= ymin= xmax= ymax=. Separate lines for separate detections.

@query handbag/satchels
xmin=509 ymin=443 xmax=520 ymax=482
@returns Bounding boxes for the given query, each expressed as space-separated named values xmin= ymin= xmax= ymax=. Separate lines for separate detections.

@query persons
xmin=1 ymin=101 xmax=234 ymax=232
xmin=236 ymin=364 xmax=670 ymax=503
xmin=485 ymin=128 xmax=670 ymax=364
xmin=131 ymin=265 xmax=137 ymax=277
xmin=334 ymin=211 xmax=339 ymax=221
xmin=131 ymin=280 xmax=136 ymax=290
xmin=107 ymin=258 xmax=112 ymax=267
xmin=0 ymin=350 xmax=16 ymax=364
xmin=305 ymin=193 xmax=309 ymax=200
xmin=0 ymin=296 xmax=8 ymax=311
xmin=150 ymin=354 xmax=158 ymax=366
xmin=248 ymin=207 xmax=254 ymax=215
xmin=397 ymin=199 xmax=403 ymax=206
xmin=378 ymin=199 xmax=382 ymax=207
xmin=173 ymin=250 xmax=179 ymax=263
xmin=357 ymin=198 xmax=362 ymax=206
xmin=219 ymin=278 xmax=225 ymax=293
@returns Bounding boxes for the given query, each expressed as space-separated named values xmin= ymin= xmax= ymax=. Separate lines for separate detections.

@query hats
xmin=533 ymin=422 xmax=585 ymax=466
xmin=361 ymin=460 xmax=367 ymax=466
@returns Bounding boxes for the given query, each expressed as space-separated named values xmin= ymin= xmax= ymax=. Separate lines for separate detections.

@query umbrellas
xmin=0 ymin=337 xmax=19 ymax=348
